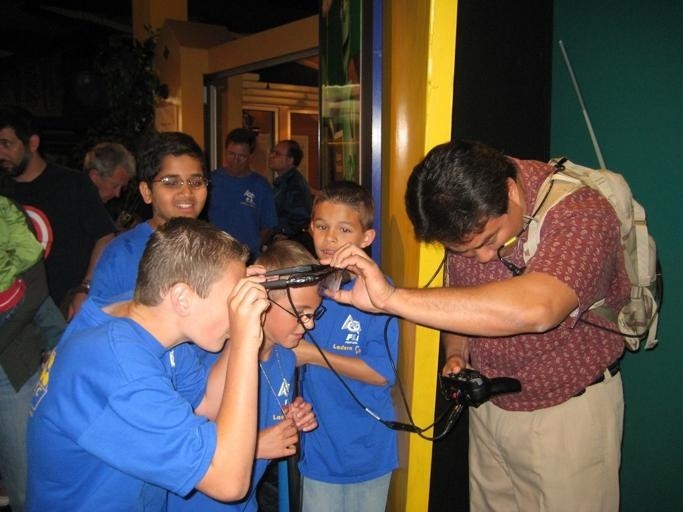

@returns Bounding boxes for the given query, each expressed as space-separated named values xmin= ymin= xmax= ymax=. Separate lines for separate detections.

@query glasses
xmin=226 ymin=151 xmax=249 ymax=160
xmin=268 ymin=296 xmax=327 ymax=324
xmin=150 ymin=176 xmax=212 ymax=190
xmin=271 ymin=148 xmax=288 ymax=157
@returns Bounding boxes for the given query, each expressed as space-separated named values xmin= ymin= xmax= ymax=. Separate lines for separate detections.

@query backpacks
xmin=522 ymin=157 xmax=664 ymax=352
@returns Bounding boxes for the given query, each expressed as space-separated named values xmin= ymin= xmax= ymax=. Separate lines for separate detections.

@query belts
xmin=573 ymin=360 xmax=621 ymax=397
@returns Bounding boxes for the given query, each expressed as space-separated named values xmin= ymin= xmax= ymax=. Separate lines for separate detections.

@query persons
xmin=1 ymin=104 xmax=401 ymax=512
xmin=315 ymin=134 xmax=636 ymax=512
